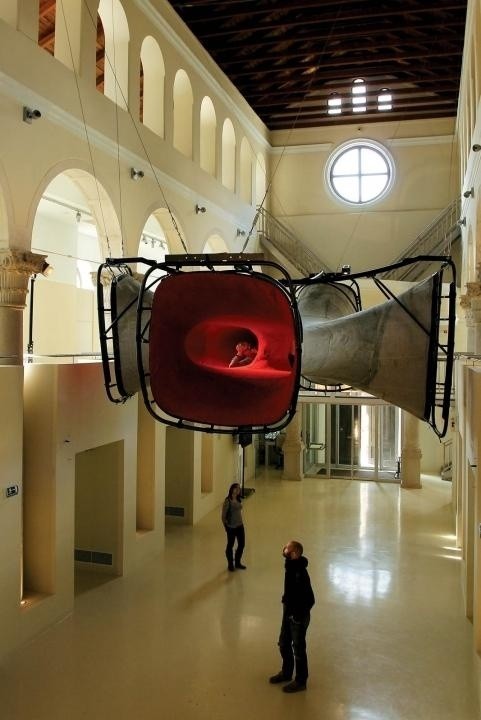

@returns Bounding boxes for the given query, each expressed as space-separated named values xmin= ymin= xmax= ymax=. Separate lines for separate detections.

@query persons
xmin=269 ymin=540 xmax=316 ymax=693
xmin=222 ymin=483 xmax=256 ymax=572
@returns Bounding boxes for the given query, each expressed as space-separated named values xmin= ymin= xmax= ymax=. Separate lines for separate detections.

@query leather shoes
xmin=235 ymin=563 xmax=246 ymax=569
xmin=282 ymin=681 xmax=306 ymax=693
xmin=269 ymin=674 xmax=293 ymax=684
xmin=228 ymin=564 xmax=235 ymax=572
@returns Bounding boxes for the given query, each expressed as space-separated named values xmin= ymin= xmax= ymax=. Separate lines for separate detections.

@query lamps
xmin=23 ymin=105 xmax=42 ymax=124
xmin=464 ymin=187 xmax=474 ymax=199
xmin=472 ymin=144 xmax=481 ymax=151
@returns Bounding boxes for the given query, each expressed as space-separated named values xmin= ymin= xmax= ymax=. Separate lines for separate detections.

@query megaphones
xmin=132 ymin=248 xmax=302 ymax=439
xmin=301 ymin=254 xmax=456 ymax=441
xmin=297 ymin=278 xmax=363 ymax=392
xmin=95 ymin=257 xmax=155 ymax=406
